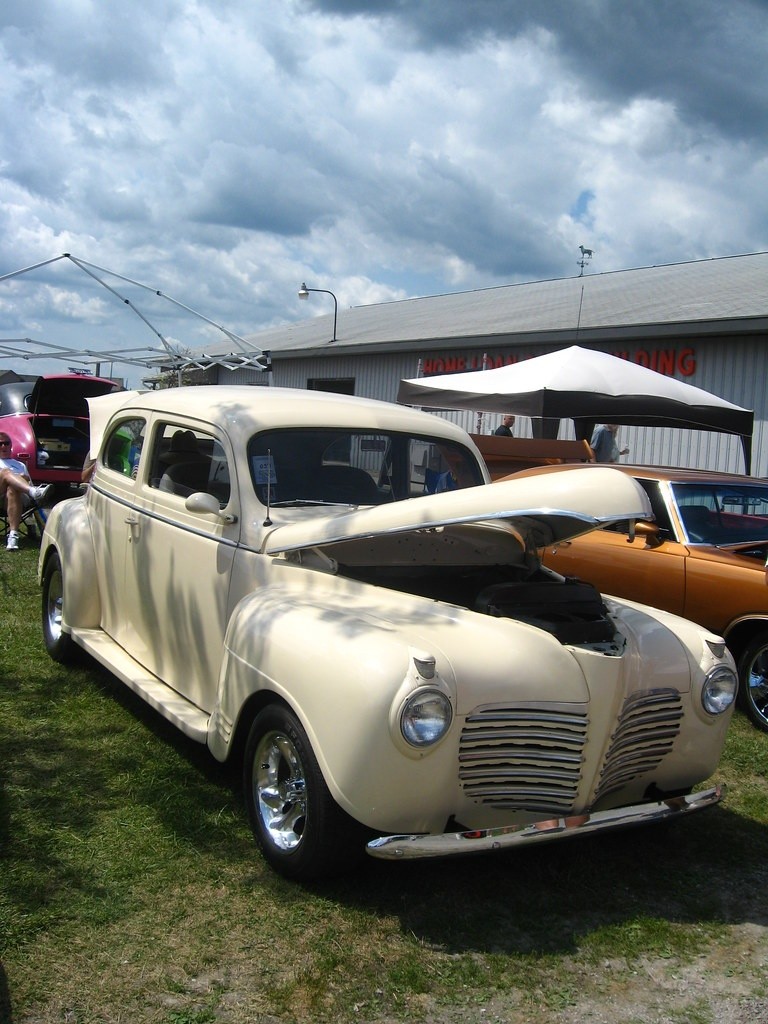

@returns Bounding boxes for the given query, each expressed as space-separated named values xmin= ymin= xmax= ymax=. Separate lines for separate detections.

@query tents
xmin=395 ymin=346 xmax=754 ymax=516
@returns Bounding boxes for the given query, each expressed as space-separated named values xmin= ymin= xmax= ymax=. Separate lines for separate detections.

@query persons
xmin=494 ymin=413 xmax=515 ymax=437
xmin=82 ymin=450 xmax=96 ymax=482
xmin=0 ymin=431 xmax=53 ymax=551
xmin=587 ymin=423 xmax=630 ymax=464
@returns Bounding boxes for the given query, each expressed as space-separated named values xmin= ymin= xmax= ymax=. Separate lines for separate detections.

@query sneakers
xmin=7 ymin=534 xmax=19 ymax=550
xmin=34 ymin=484 xmax=53 ymax=508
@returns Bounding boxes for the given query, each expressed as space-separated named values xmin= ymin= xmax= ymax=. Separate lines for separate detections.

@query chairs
xmin=207 ymin=465 xmax=306 ymax=507
xmin=155 ymin=430 xmax=204 ymax=487
xmin=162 ymin=430 xmax=229 ymax=502
xmin=309 ymin=464 xmax=381 ymax=504
xmin=681 ymin=505 xmax=711 ymax=530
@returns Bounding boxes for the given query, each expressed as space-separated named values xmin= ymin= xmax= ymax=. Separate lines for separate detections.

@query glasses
xmin=0 ymin=441 xmax=10 ymax=446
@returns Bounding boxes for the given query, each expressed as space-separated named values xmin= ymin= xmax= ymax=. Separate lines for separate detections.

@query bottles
xmin=27 ymin=515 xmax=37 ymax=545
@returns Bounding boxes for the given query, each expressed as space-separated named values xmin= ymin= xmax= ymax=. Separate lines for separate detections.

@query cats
xmin=579 ymin=245 xmax=596 ymax=259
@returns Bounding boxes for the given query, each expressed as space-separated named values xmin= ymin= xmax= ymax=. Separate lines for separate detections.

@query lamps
xmin=298 ymin=282 xmax=337 ymax=341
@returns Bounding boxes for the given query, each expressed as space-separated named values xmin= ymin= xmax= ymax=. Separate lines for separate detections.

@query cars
xmin=0 ymin=372 xmax=141 ymax=484
xmin=36 ymin=383 xmax=741 ymax=886
xmin=435 ymin=430 xmax=768 ymax=734
